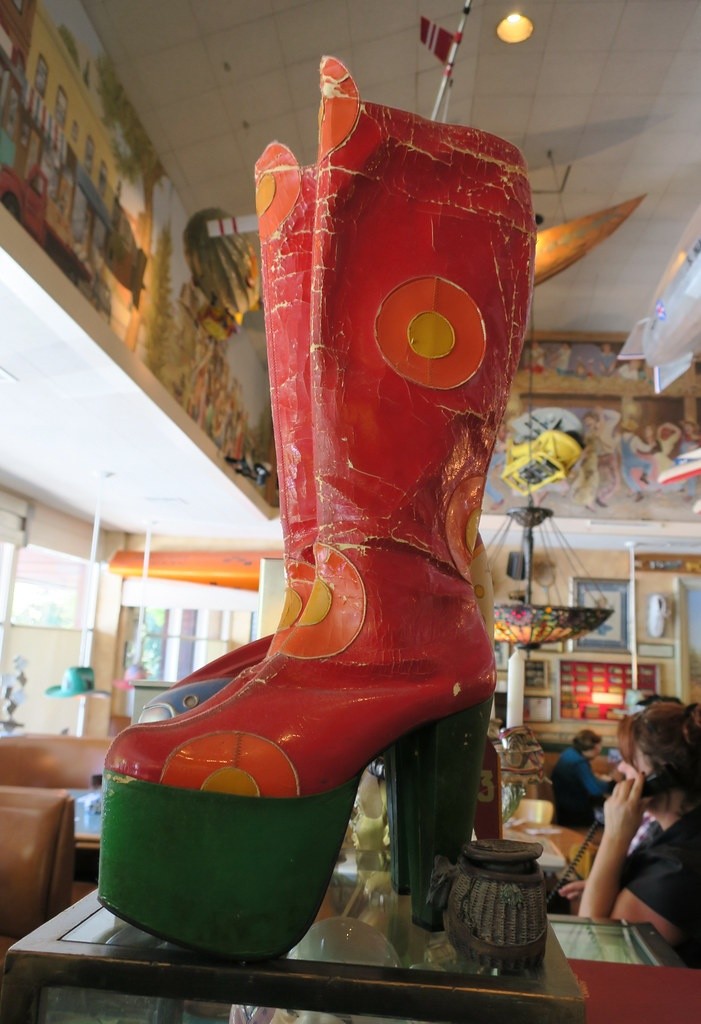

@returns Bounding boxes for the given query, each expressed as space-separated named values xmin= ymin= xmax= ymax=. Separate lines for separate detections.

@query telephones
xmin=593 ymin=764 xmax=684 ymax=826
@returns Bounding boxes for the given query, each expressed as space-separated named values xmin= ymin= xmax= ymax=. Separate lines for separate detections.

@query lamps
xmin=497 ymin=10 xmax=535 ymax=45
xmin=484 ymin=297 xmax=617 ymax=653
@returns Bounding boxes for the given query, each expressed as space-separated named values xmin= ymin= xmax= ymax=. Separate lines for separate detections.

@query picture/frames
xmin=568 ymin=576 xmax=632 ymax=654
xmin=524 ymin=695 xmax=553 ymax=724
xmin=494 ymin=640 xmax=511 ymax=672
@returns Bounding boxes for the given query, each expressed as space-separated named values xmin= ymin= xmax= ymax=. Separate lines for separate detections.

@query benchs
xmin=0 ymin=735 xmax=115 ymax=883
xmin=0 ymin=786 xmax=76 ymax=1024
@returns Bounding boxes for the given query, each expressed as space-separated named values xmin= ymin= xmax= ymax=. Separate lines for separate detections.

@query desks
xmin=0 ymin=848 xmax=588 ymax=1024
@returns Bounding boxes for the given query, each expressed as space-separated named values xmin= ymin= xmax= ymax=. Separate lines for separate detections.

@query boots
xmin=96 ymin=52 xmax=538 ymax=949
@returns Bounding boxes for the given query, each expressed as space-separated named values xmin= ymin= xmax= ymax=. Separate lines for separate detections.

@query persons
xmin=549 ymin=730 xmax=614 ymax=829
xmin=558 ymin=693 xmax=700 ymax=960
